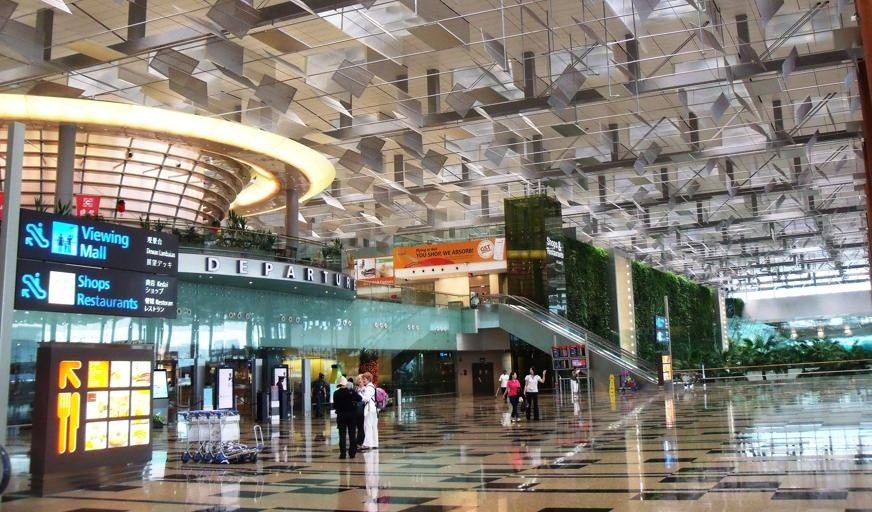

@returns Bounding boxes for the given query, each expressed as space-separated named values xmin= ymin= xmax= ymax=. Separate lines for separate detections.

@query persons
xmin=277 ymin=376 xmax=284 ymax=420
xmin=570 ymin=367 xmax=580 ymax=393
xmin=507 ymin=371 xmax=522 ymax=422
xmin=333 ymin=363 xmax=379 ymax=459
xmin=498 ymin=369 xmax=510 ymax=403
xmin=523 ymin=367 xmax=547 ymax=420
xmin=310 ymin=374 xmax=330 ymax=417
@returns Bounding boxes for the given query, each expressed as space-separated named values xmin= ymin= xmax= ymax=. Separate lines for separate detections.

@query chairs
xmin=277 ymin=246 xmax=297 ymax=264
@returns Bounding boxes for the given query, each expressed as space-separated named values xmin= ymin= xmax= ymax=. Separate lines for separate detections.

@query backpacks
xmin=366 ymin=385 xmax=388 ymax=410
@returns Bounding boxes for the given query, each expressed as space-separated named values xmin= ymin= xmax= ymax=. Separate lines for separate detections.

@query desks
xmin=273 ymin=249 xmax=286 ymax=258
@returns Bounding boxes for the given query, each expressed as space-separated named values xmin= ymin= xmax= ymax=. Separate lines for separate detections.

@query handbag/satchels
xmin=518 ymin=387 xmax=529 ymax=412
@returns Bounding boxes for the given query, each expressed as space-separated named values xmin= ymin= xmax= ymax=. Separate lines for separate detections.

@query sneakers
xmin=510 ymin=417 xmax=521 ymax=422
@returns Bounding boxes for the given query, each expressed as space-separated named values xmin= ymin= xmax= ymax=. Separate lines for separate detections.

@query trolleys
xmin=176 ymin=409 xmax=265 ymax=464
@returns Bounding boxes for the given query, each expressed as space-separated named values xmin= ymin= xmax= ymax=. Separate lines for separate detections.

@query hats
xmin=336 ymin=375 xmax=347 ymax=386
